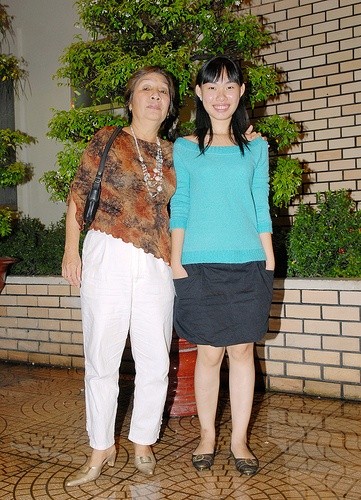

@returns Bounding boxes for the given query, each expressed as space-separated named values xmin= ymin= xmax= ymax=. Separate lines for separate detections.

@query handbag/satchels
xmin=82 ymin=125 xmax=124 ymax=234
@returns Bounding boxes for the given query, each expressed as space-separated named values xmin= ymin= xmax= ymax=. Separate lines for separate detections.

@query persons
xmin=61 ymin=66 xmax=270 ymax=487
xmin=170 ymin=56 xmax=276 ymax=476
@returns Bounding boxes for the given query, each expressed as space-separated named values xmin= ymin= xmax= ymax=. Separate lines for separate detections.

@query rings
xmin=67 ymin=275 xmax=70 ymax=278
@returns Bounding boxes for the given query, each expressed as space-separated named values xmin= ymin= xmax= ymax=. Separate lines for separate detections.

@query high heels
xmin=134 ymin=452 xmax=156 ymax=475
xmin=64 ymin=447 xmax=116 ymax=487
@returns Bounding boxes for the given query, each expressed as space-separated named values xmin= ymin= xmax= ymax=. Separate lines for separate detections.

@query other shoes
xmin=191 ymin=440 xmax=216 ymax=471
xmin=229 ymin=442 xmax=259 ymax=476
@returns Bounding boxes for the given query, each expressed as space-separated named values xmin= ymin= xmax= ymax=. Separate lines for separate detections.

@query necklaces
xmin=129 ymin=124 xmax=163 ymax=197
